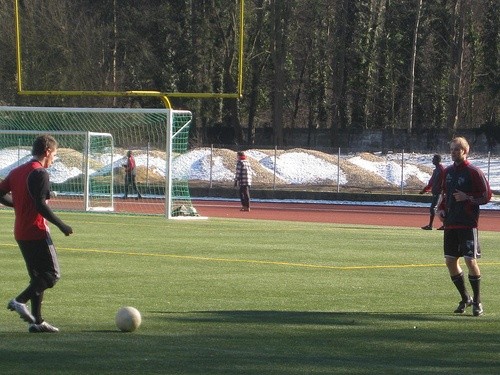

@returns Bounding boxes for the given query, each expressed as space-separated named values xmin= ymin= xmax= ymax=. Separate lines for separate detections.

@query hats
xmin=237 ymin=151 xmax=244 ymax=156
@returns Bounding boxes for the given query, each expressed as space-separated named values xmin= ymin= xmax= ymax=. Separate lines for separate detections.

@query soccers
xmin=116 ymin=306 xmax=142 ymax=334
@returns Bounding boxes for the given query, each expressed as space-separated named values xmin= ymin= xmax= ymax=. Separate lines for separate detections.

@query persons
xmin=419 ymin=155 xmax=446 ymax=230
xmin=0 ymin=135 xmax=72 ymax=332
xmin=122 ymin=151 xmax=142 ymax=200
xmin=234 ymin=152 xmax=252 ymax=211
xmin=436 ymin=137 xmax=492 ymax=316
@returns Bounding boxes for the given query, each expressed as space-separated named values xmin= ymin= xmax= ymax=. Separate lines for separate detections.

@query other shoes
xmin=422 ymin=225 xmax=432 ymax=230
xmin=437 ymin=226 xmax=444 ymax=230
xmin=135 ymin=195 xmax=141 ymax=199
xmin=121 ymin=197 xmax=127 ymax=199
xmin=240 ymin=207 xmax=250 ymax=211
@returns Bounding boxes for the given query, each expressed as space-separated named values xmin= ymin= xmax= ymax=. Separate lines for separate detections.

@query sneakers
xmin=455 ymin=297 xmax=473 ymax=313
xmin=473 ymin=301 xmax=483 ymax=316
xmin=7 ymin=298 xmax=36 ymax=324
xmin=29 ymin=320 xmax=59 ymax=332
